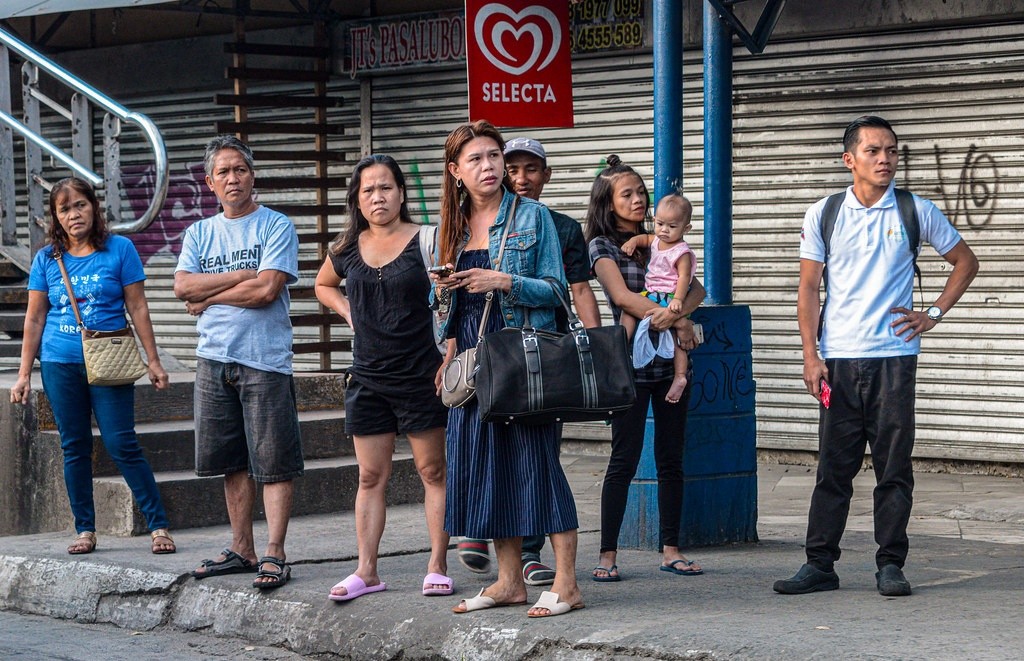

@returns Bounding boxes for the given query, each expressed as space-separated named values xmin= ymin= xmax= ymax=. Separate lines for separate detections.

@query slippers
xmin=660 ymin=559 xmax=703 ymax=575
xmin=451 ymin=587 xmax=527 ymax=613
xmin=66 ymin=530 xmax=97 ymax=554
xmin=150 ymin=529 xmax=176 ymax=553
xmin=422 ymin=573 xmax=453 ymax=596
xmin=527 ymin=590 xmax=586 ymax=617
xmin=456 ymin=537 xmax=491 ymax=574
xmin=253 ymin=556 xmax=291 ymax=588
xmin=592 ymin=564 xmax=620 ymax=581
xmin=522 ymin=560 xmax=555 ymax=586
xmin=194 ymin=548 xmax=258 ymax=579
xmin=328 ymin=574 xmax=386 ymax=600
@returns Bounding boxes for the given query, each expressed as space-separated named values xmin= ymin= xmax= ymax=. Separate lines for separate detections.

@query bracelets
xmin=673 ymin=298 xmax=683 ymax=301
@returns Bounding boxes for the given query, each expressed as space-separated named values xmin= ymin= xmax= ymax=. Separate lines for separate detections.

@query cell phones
xmin=428 ymin=265 xmax=463 ymax=281
xmin=818 ymin=376 xmax=833 ymax=409
xmin=677 ymin=324 xmax=704 ymax=346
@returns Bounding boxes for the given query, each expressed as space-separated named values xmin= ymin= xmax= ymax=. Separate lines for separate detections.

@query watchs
xmin=927 ymin=305 xmax=943 ymax=323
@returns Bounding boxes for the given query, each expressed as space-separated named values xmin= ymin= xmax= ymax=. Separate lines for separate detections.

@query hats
xmin=504 ymin=137 xmax=548 ymax=167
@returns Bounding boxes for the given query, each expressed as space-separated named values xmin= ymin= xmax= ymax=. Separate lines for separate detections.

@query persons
xmin=315 ymin=154 xmax=454 ymax=601
xmin=772 ymin=116 xmax=980 ymax=597
xmin=173 ymin=137 xmax=299 ymax=588
xmin=429 ymin=122 xmax=585 ymax=617
xmin=9 ymin=177 xmax=178 ymax=554
xmin=617 ymin=194 xmax=697 ymax=403
xmin=458 ymin=137 xmax=604 ymax=587
xmin=586 ymin=154 xmax=704 ymax=582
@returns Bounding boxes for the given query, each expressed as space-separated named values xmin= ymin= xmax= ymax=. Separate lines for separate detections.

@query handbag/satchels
xmin=440 ymin=347 xmax=478 ymax=409
xmin=474 ymin=321 xmax=638 ymax=422
xmin=80 ymin=327 xmax=148 ymax=387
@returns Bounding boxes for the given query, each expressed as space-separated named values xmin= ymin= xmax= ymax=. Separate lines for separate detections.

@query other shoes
xmin=875 ymin=564 xmax=913 ymax=596
xmin=773 ymin=563 xmax=840 ymax=594
